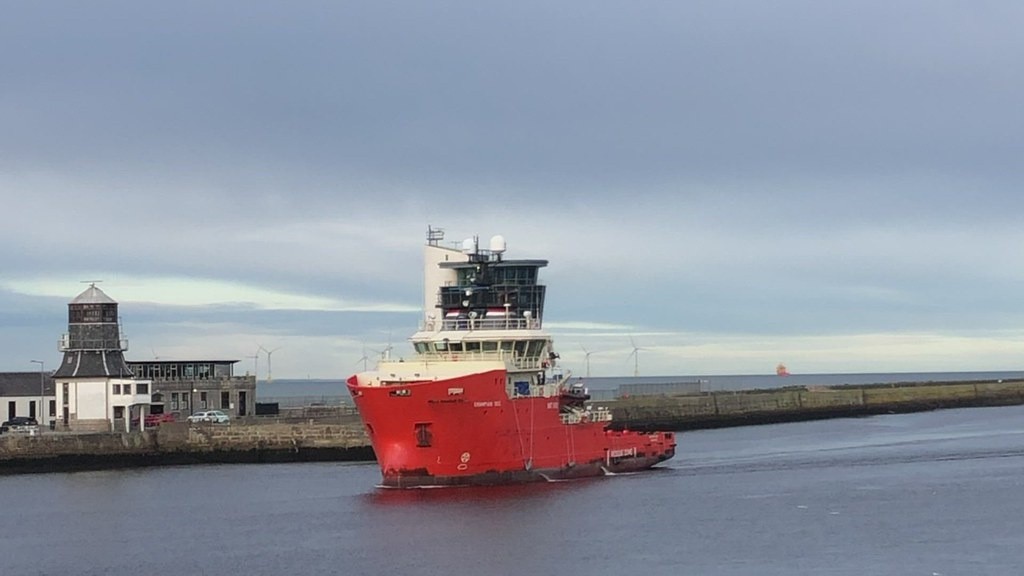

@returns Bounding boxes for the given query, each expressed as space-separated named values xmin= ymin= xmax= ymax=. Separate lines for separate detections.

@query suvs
xmin=2 ymin=417 xmax=38 ymax=427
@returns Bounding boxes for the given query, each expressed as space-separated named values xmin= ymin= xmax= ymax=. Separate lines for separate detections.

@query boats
xmin=344 ymin=220 xmax=678 ymax=488
xmin=776 ymin=362 xmax=791 ymax=377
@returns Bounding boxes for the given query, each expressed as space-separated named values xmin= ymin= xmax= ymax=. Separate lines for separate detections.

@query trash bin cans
xmin=514 ymin=381 xmax=530 ymax=396
xmin=50 ymin=421 xmax=55 ymax=429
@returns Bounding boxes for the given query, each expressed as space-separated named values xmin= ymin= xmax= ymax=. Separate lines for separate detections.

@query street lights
xmin=31 ymin=360 xmax=44 ymax=424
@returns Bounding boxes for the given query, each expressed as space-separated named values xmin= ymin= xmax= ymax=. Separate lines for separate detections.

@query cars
xmin=131 ymin=412 xmax=178 ymax=427
xmin=186 ymin=411 xmax=217 ymax=423
xmin=206 ymin=410 xmax=230 ymax=423
